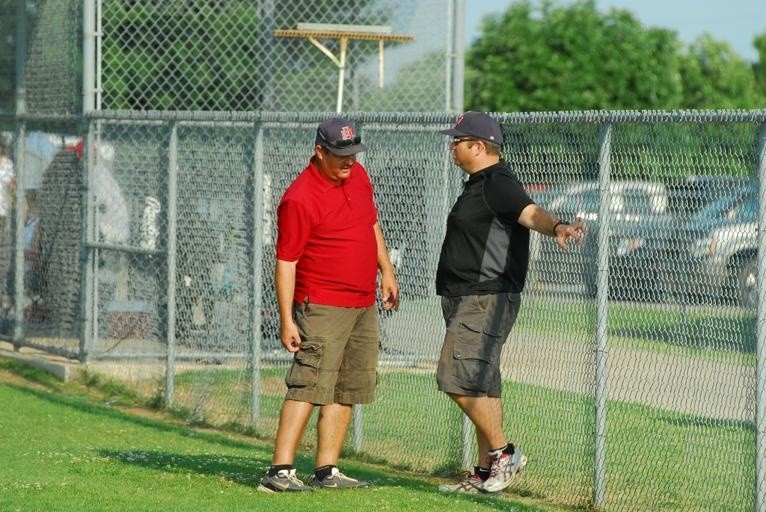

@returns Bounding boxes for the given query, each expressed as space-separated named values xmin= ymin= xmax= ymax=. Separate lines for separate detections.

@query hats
xmin=315 ymin=118 xmax=367 ymax=157
xmin=439 ymin=112 xmax=504 ymax=146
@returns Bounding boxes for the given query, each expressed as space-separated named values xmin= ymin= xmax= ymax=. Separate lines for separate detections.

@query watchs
xmin=553 ymin=220 xmax=570 ymax=237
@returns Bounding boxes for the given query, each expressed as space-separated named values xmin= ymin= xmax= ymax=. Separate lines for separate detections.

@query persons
xmin=436 ymin=113 xmax=589 ymax=494
xmin=1 ymin=88 xmax=58 ymax=322
xmin=256 ymin=118 xmax=400 ymax=495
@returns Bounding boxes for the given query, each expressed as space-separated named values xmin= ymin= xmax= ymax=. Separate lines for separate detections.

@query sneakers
xmin=256 ymin=467 xmax=314 ymax=496
xmin=438 ymin=448 xmax=528 ymax=499
xmin=311 ymin=467 xmax=373 ymax=490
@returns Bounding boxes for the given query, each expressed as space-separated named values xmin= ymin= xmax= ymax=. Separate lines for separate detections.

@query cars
xmin=524 ymin=176 xmax=760 ymax=310
